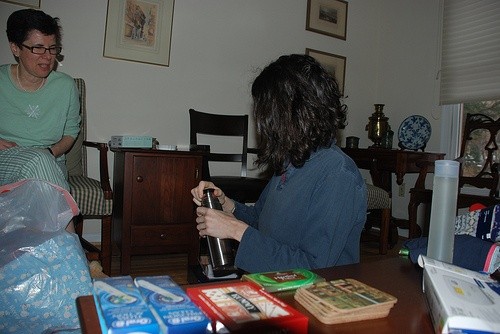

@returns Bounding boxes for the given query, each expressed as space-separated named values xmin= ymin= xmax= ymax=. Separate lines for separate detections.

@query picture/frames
xmin=0 ymin=0 xmax=40 ymax=9
xmin=305 ymin=0 xmax=348 ymax=40
xmin=305 ymin=48 xmax=346 ymax=96
xmin=102 ymin=0 xmax=175 ymax=67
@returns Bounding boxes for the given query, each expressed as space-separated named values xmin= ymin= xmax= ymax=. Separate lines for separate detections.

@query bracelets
xmin=46 ymin=147 xmax=54 ymax=157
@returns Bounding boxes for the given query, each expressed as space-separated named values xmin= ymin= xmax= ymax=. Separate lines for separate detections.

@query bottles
xmin=200 ymin=188 xmax=236 ymax=277
xmin=428 ymin=159 xmax=460 ymax=265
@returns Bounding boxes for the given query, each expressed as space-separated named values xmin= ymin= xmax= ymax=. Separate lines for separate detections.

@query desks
xmin=339 ymin=148 xmax=446 ymax=251
xmin=76 ymin=254 xmax=435 ymax=334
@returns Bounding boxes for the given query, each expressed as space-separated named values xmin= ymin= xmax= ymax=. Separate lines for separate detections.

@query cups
xmin=346 ymin=136 xmax=359 ymax=149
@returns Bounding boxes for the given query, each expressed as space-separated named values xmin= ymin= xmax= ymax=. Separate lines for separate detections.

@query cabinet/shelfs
xmin=110 ymin=147 xmax=206 ymax=284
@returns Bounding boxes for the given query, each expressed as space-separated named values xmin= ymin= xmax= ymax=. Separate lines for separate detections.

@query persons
xmin=0 ymin=8 xmax=82 ymax=266
xmin=190 ymin=53 xmax=369 ymax=278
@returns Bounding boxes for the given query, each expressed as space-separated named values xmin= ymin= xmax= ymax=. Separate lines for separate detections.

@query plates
xmin=399 ymin=115 xmax=432 ymax=150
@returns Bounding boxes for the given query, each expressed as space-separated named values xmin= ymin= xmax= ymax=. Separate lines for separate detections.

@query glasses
xmin=22 ymin=44 xmax=62 ymax=55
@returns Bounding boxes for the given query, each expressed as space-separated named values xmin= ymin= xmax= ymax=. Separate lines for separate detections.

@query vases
xmin=366 ymin=104 xmax=392 ymax=148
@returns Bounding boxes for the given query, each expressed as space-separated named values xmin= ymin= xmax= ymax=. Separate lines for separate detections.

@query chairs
xmin=67 ymin=79 xmax=112 ymax=277
xmin=408 ymin=113 xmax=500 ymax=239
xmin=189 ymin=108 xmax=268 ymax=253
